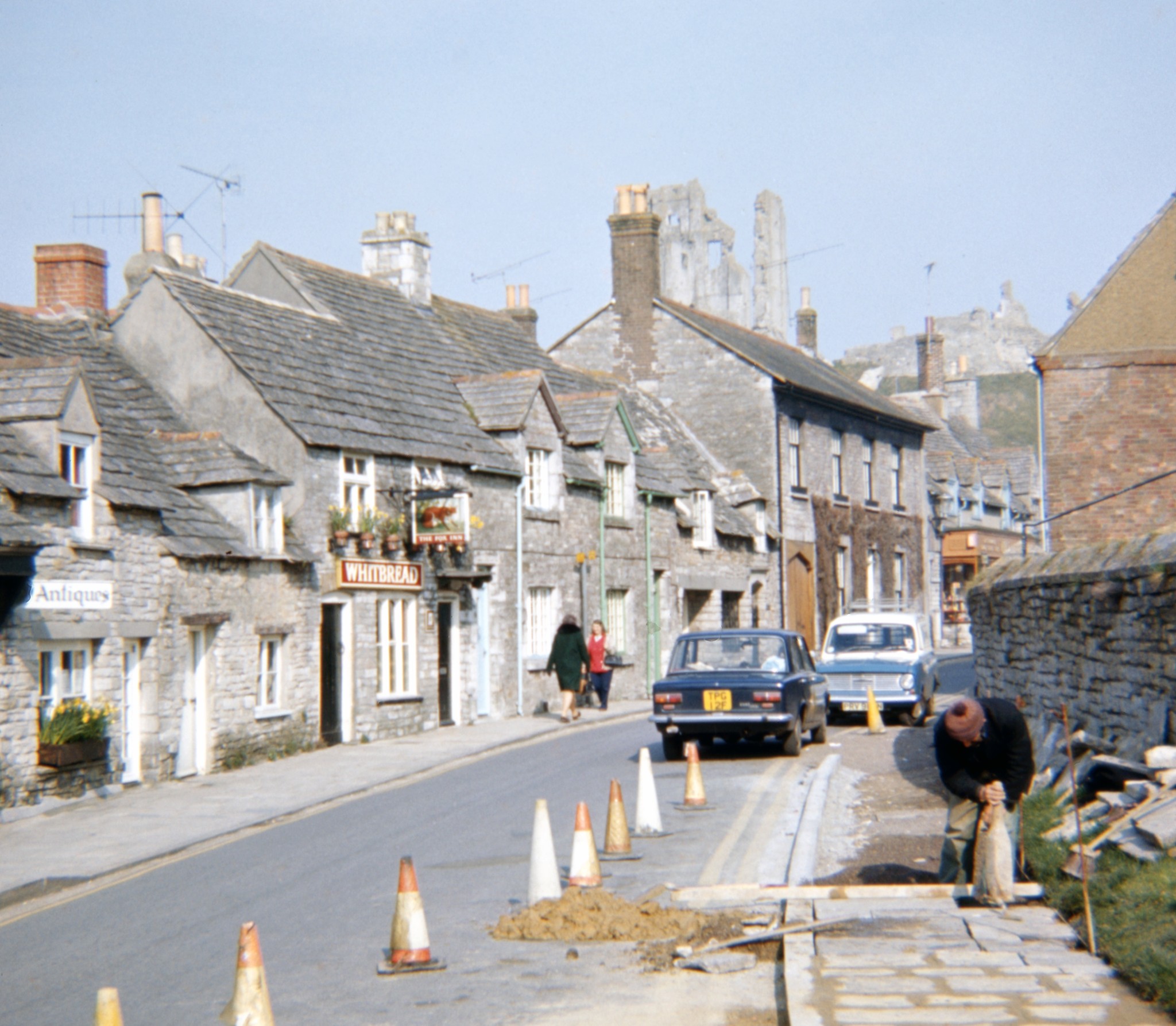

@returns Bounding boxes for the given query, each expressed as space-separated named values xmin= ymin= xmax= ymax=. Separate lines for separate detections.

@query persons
xmin=581 ymin=620 xmax=615 ymax=710
xmin=934 ymin=698 xmax=1035 ymax=885
xmin=546 ymin=615 xmax=590 ymax=722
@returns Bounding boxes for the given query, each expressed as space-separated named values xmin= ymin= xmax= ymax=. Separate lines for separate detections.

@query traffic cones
xmin=94 ymin=987 xmax=123 ymax=1026
xmin=864 ymin=686 xmax=891 ymax=735
xmin=620 ymin=747 xmax=674 ymax=838
xmin=562 ymin=803 xmax=608 ymax=889
xmin=584 ymin=778 xmax=641 ymax=860
xmin=675 ymin=742 xmax=716 ymax=812
xmin=218 ymin=920 xmax=276 ymax=1026
xmin=526 ymin=797 xmax=564 ymax=904
xmin=375 ymin=853 xmax=446 ymax=973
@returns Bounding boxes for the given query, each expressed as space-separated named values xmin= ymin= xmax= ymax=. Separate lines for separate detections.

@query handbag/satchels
xmin=604 ymin=650 xmax=622 ymax=666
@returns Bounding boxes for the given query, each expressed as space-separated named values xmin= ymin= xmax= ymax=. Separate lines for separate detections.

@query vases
xmin=386 ymin=534 xmax=399 ymax=550
xmin=360 ymin=532 xmax=375 ymax=549
xmin=335 ymin=530 xmax=349 ymax=547
xmin=39 ymin=737 xmax=111 ymax=767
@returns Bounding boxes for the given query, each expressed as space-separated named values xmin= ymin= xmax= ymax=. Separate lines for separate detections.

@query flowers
xmin=329 ymin=504 xmax=353 ymax=530
xmin=382 ymin=513 xmax=406 ymax=534
xmin=40 ymin=696 xmax=119 ymax=744
xmin=358 ymin=505 xmax=384 ymax=530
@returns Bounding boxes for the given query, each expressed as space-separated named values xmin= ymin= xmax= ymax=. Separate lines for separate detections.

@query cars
xmin=648 ymin=629 xmax=828 ymax=754
xmin=813 ymin=611 xmax=943 ymax=718
